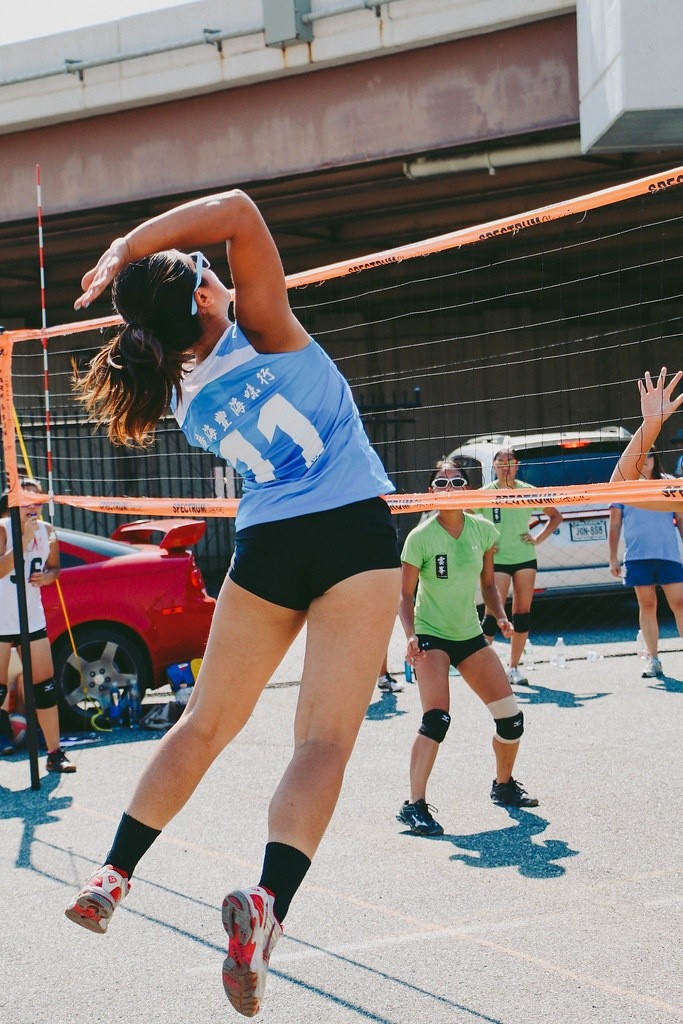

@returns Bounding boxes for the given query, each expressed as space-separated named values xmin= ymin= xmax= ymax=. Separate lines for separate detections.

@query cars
xmin=20 ymin=514 xmax=217 ymax=735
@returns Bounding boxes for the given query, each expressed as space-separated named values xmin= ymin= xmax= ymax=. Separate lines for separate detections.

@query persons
xmin=396 ymin=460 xmax=539 ymax=836
xmin=465 ymin=448 xmax=563 ymax=685
xmin=609 ymin=365 xmax=683 ymax=677
xmin=0 ymin=464 xmax=77 ymax=774
xmin=64 ymin=188 xmax=402 ymax=1017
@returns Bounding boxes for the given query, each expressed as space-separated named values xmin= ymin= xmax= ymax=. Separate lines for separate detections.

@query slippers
xmin=647 ymin=450 xmax=655 ymax=457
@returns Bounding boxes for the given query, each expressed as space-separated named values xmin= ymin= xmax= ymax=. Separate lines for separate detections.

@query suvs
xmin=415 ymin=424 xmax=636 ymax=617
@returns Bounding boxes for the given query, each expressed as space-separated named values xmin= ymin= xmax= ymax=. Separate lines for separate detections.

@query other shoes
xmin=0 ymin=736 xmax=16 ymax=755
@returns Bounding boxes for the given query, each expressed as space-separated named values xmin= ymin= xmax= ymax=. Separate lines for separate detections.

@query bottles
xmin=555 ymin=637 xmax=567 ymax=668
xmin=110 ymin=682 xmax=125 ymax=730
xmin=523 ymin=639 xmax=534 ymax=671
xmin=636 ymin=630 xmax=649 ymax=660
xmin=176 ymin=684 xmax=190 ymax=714
xmin=128 ymin=679 xmax=143 ymax=729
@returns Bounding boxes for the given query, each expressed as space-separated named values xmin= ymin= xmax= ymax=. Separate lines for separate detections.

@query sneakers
xmin=64 ymin=865 xmax=131 ymax=934
xmin=642 ymin=658 xmax=666 ymax=678
xmin=378 ymin=671 xmax=404 ymax=692
xmin=489 ymin=776 xmax=539 ymax=808
xmin=221 ymin=886 xmax=283 ymax=1017
xmin=395 ymin=798 xmax=444 ymax=836
xmin=506 ymin=667 xmax=531 ymax=685
xmin=46 ymin=747 xmax=77 ymax=773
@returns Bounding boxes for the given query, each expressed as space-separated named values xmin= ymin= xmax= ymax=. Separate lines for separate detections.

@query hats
xmin=670 ymin=429 xmax=683 ymax=443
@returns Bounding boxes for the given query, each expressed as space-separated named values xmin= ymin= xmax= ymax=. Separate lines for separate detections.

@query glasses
xmin=494 ymin=459 xmax=518 ymax=469
xmin=431 ymin=477 xmax=469 ymax=490
xmin=188 ymin=251 xmax=211 ymax=316
